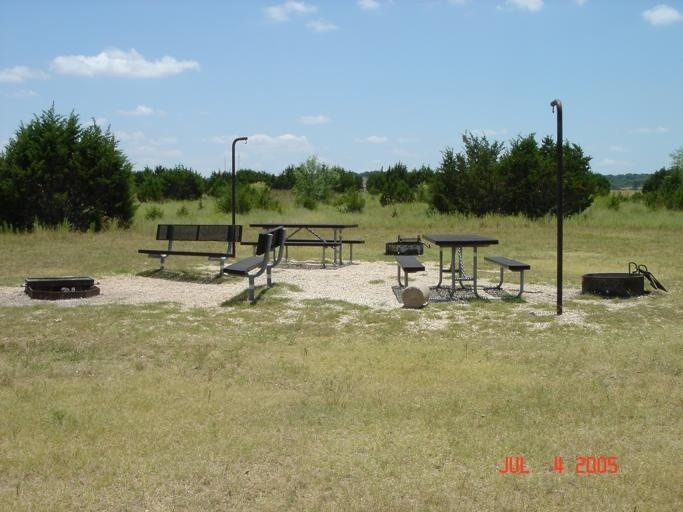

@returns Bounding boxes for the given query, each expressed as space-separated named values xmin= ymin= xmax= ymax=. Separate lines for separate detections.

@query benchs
xmin=239 ymin=224 xmax=365 ymax=267
xmin=394 ymin=234 xmax=530 ymax=299
xmin=138 ymin=224 xmax=286 ymax=303
